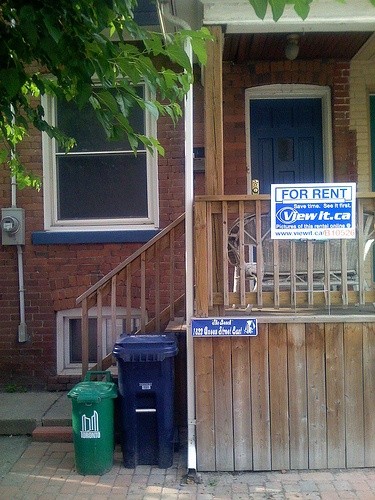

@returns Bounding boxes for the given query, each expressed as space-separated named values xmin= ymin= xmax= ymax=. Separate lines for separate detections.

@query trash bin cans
xmin=66 ymin=369 xmax=119 ymax=475
xmin=112 ymin=331 xmax=180 ymax=468
